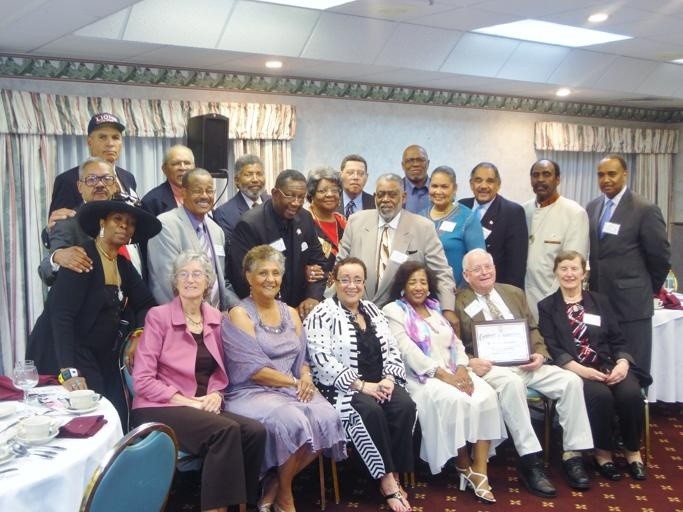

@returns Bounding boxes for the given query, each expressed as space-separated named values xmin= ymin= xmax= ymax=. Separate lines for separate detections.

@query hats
xmin=88 ymin=114 xmax=130 ymax=134
xmin=77 ymin=192 xmax=163 ymax=246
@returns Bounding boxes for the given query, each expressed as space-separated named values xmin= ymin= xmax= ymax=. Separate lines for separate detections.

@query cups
xmin=21 ymin=416 xmax=60 ymax=436
xmin=70 ymin=390 xmax=100 ymax=407
xmin=20 ymin=360 xmax=35 ymax=366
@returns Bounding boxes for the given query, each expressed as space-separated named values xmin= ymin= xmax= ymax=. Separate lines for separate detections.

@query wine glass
xmin=12 ymin=366 xmax=39 ymax=403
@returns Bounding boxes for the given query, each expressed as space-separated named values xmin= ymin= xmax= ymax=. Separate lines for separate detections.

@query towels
xmin=0 ymin=375 xmax=24 ymax=400
xmin=39 ymin=375 xmax=60 ymax=386
xmin=653 ymin=288 xmax=683 ymax=310
xmin=58 ymin=415 xmax=107 ymax=439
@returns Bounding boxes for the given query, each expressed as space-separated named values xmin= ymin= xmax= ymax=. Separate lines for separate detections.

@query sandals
xmin=454 ymin=466 xmax=498 ymax=503
xmin=379 ymin=479 xmax=404 ymax=500
xmin=375 ymin=480 xmax=421 ymax=512
xmin=452 ymin=463 xmax=492 ymax=491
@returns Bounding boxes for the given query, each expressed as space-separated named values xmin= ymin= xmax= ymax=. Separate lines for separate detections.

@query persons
xmin=26 ymin=113 xmax=672 ymax=510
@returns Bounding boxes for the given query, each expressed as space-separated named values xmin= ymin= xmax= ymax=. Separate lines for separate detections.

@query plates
xmin=1 ymin=446 xmax=15 ymax=465
xmin=16 ymin=430 xmax=60 ymax=444
xmin=65 ymin=404 xmax=98 ymax=414
xmin=0 ymin=400 xmax=17 ymax=418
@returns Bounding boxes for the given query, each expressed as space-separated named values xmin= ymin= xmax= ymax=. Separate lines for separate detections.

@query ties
xmin=483 ymin=294 xmax=505 ymax=319
xmin=194 ymin=222 xmax=221 ymax=310
xmin=600 ymin=200 xmax=615 ymax=236
xmin=345 ymin=202 xmax=355 ymax=219
xmin=250 ymin=200 xmax=260 ymax=209
xmin=376 ymin=225 xmax=391 ymax=290
xmin=474 ymin=204 xmax=484 ymax=216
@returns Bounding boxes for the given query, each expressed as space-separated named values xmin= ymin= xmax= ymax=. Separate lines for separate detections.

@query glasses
xmin=185 ymin=186 xmax=220 ymax=199
xmin=278 ymin=189 xmax=309 ymax=204
xmin=335 ymin=277 xmax=367 ymax=287
xmin=174 ymin=272 xmax=207 ymax=282
xmin=315 ymin=187 xmax=343 ymax=196
xmin=465 ymin=266 xmax=500 ymax=274
xmin=84 ymin=175 xmax=115 ymax=189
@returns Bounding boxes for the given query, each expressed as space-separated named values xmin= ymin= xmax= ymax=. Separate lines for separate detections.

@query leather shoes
xmin=274 ymin=498 xmax=297 ymax=512
xmin=519 ymin=464 xmax=556 ymax=500
xmin=562 ymin=451 xmax=592 ymax=492
xmin=622 ymin=456 xmax=648 ymax=482
xmin=255 ymin=496 xmax=273 ymax=511
xmin=588 ymin=454 xmax=618 ymax=482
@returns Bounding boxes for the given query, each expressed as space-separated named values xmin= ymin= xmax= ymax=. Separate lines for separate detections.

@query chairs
xmin=79 ymin=421 xmax=178 ymax=512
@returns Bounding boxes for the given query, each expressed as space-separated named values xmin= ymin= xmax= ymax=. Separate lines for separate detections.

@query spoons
xmin=8 ymin=440 xmax=53 ymax=464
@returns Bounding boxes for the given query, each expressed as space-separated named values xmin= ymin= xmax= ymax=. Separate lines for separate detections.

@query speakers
xmin=186 ymin=113 xmax=229 ymax=178
